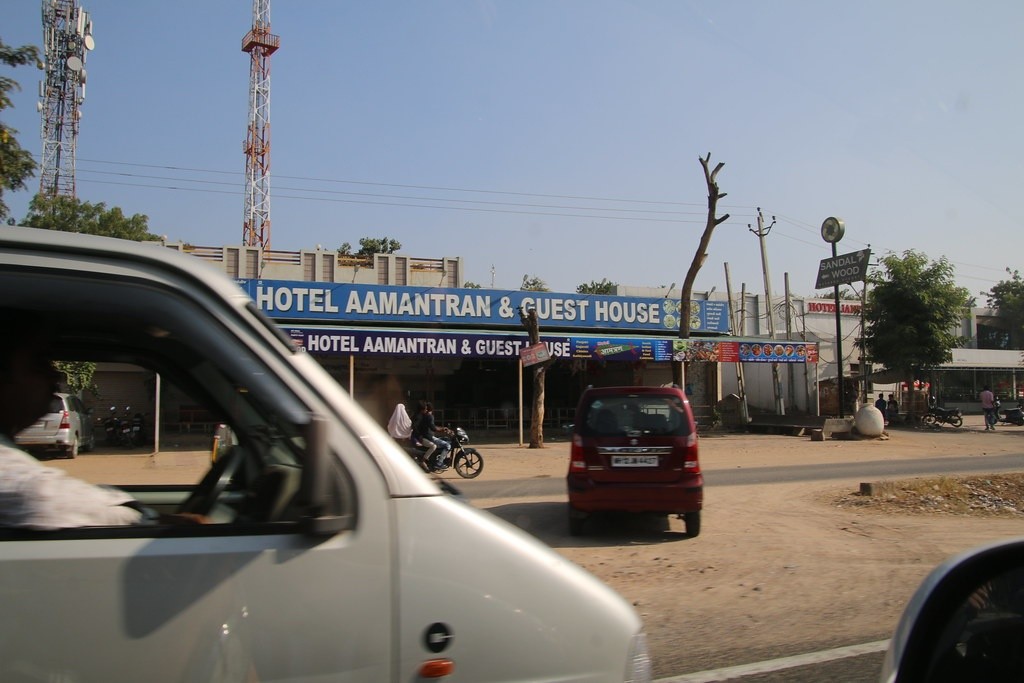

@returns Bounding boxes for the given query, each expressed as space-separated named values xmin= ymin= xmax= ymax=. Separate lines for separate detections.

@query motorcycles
xmin=96 ymin=405 xmax=146 ymax=449
xmin=395 ymin=422 xmax=484 ymax=479
xmin=988 ymin=396 xmax=1024 ymax=427
xmin=921 ymin=397 xmax=963 ymax=428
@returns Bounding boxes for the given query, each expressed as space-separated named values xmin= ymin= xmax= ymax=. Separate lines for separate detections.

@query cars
xmin=563 ymin=382 xmax=704 ymax=537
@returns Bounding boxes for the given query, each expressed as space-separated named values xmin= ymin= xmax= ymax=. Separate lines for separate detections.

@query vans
xmin=0 ymin=224 xmax=654 ymax=683
xmin=14 ymin=390 xmax=95 ymax=457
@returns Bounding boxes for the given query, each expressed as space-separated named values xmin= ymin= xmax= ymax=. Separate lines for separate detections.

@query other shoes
xmin=432 ymin=462 xmax=449 ymax=470
xmin=420 ymin=458 xmax=431 ymax=472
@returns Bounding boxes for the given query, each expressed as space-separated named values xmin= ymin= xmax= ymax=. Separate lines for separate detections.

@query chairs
xmin=596 ymin=409 xmax=619 ymax=433
xmin=642 ymin=412 xmax=665 ymax=432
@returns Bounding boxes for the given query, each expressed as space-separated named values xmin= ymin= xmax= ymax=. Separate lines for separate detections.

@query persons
xmin=886 ymin=393 xmax=898 ymax=419
xmin=875 ymin=393 xmax=886 ymax=417
xmin=0 ymin=274 xmax=205 ymax=527
xmin=980 ymin=384 xmax=995 ymax=430
xmin=411 ymin=400 xmax=451 ymax=471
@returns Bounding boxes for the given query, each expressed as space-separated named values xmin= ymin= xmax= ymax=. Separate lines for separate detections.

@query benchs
xmin=745 ymin=415 xmax=833 ymax=437
xmin=810 ymin=418 xmax=854 ymax=442
